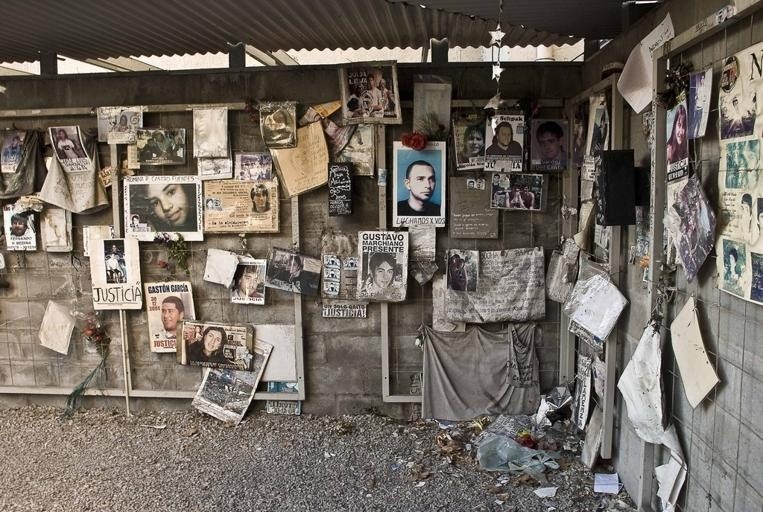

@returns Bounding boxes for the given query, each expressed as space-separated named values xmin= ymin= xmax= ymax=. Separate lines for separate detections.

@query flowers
xmin=400 ymin=113 xmax=444 ymax=150
xmin=63 ymin=310 xmax=111 ymax=413
xmin=153 ymin=231 xmax=191 ymax=274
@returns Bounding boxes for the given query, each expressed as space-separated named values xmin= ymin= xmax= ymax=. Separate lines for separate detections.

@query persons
xmin=447 ymin=254 xmax=468 ymax=290
xmin=589 ymin=111 xmax=609 ymax=154
xmin=453 ymin=118 xmax=566 ymax=212
xmin=1 ymin=101 xmax=323 ymax=367
xmin=363 ymin=254 xmax=403 ymax=301
xmin=397 ymin=159 xmax=439 ymax=215
xmin=345 ymin=69 xmax=395 ymax=120
xmin=665 ymin=56 xmax=761 ymax=303
